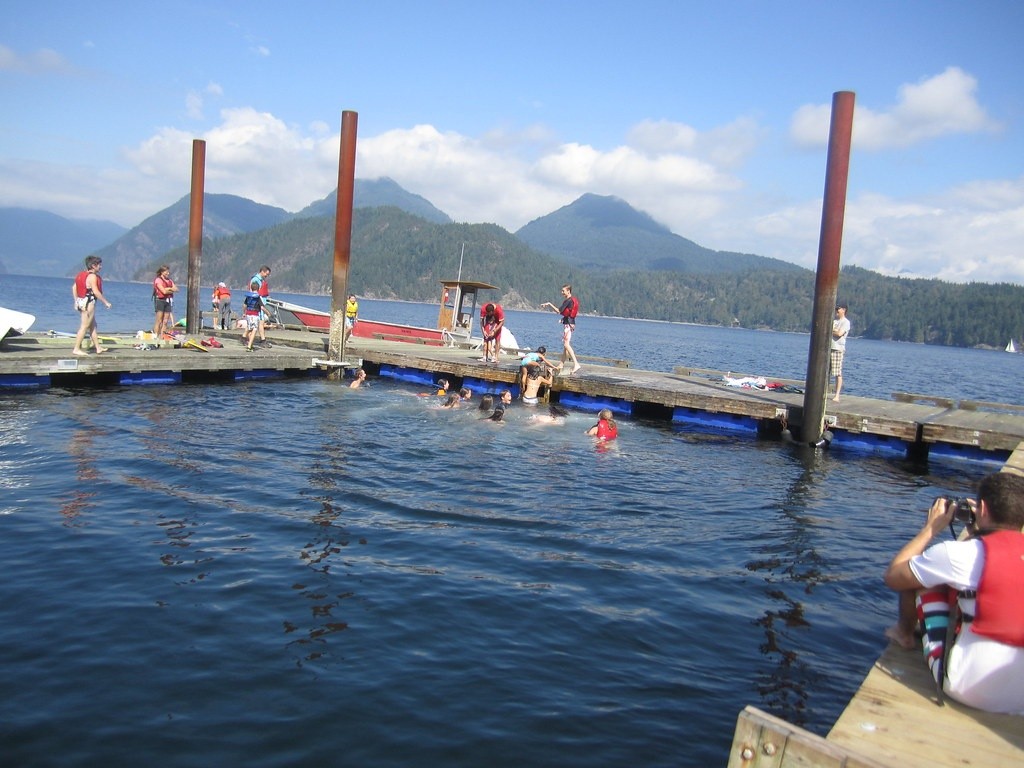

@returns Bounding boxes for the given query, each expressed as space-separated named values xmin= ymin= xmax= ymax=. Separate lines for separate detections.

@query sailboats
xmin=1005 ymin=339 xmax=1017 ymax=353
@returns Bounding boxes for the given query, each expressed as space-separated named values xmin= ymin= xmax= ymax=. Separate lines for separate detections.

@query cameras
xmin=945 ymin=496 xmax=976 ymax=525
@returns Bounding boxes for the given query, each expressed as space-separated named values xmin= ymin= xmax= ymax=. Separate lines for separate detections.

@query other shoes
xmin=215 ymin=324 xmax=222 ymax=330
xmin=259 ymin=340 xmax=272 ymax=348
xmin=491 ymin=357 xmax=496 ymax=363
xmin=224 ymin=327 xmax=227 ymax=330
xmin=477 ymin=356 xmax=486 ymax=362
xmin=247 ymin=347 xmax=255 ymax=352
xmin=240 ymin=336 xmax=248 ymax=345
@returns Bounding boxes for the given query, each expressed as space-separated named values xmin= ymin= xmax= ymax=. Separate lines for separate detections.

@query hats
xmin=835 ymin=303 xmax=847 ymax=309
xmin=219 ymin=282 xmax=226 ymax=287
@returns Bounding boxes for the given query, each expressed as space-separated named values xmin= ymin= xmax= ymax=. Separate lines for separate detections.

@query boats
xmin=265 ymin=242 xmax=532 ymax=358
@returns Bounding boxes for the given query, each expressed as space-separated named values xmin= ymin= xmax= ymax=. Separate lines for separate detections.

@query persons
xmin=585 ymin=408 xmax=618 ymax=439
xmin=345 ymin=294 xmax=359 ymax=344
xmin=541 ymin=285 xmax=581 ymax=372
xmin=242 ymin=265 xmax=272 ymax=352
xmin=479 ymin=394 xmax=493 ymax=410
xmin=498 ymin=390 xmax=512 ymax=408
xmin=829 ymin=304 xmax=851 ymax=402
xmin=884 ymin=472 xmax=1024 ymax=717
xmin=212 ymin=282 xmax=230 ymax=330
xmin=460 ymin=388 xmax=471 ymax=401
xmin=480 ymin=302 xmax=504 ymax=363
xmin=489 ymin=406 xmax=504 ymax=421
xmin=72 ymin=256 xmax=112 ymax=355
xmin=519 ymin=347 xmax=558 ymax=405
xmin=153 ymin=266 xmax=179 ymax=336
xmin=436 ymin=379 xmax=449 ymax=395
xmin=349 ymin=367 xmax=366 ymax=388
xmin=443 ymin=391 xmax=460 ymax=407
